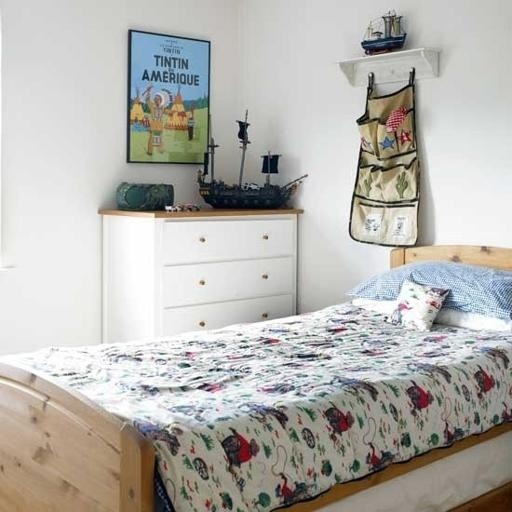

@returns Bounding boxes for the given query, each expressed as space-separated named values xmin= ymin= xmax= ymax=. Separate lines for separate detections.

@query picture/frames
xmin=127 ymin=30 xmax=211 ymax=164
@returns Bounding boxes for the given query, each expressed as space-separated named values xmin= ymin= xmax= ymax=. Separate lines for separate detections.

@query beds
xmin=0 ymin=244 xmax=511 ymax=512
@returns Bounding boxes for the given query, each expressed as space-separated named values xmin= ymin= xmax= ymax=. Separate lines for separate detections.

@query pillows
xmin=343 ymin=259 xmax=510 ymax=318
xmin=386 ymin=279 xmax=451 ymax=333
xmin=353 ymin=299 xmax=510 ymax=332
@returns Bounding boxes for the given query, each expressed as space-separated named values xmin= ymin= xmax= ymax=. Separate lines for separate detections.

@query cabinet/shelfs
xmin=99 ymin=206 xmax=302 ymax=345
